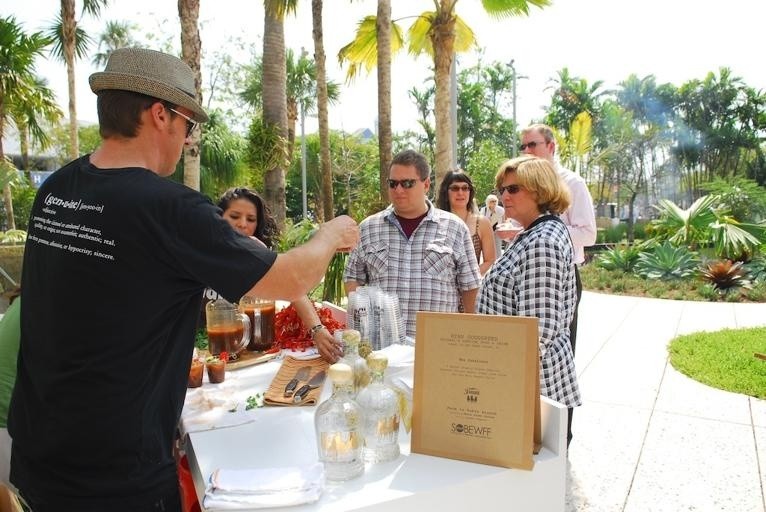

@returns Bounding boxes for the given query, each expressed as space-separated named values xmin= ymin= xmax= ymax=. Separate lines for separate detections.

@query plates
xmin=494 ymin=230 xmax=521 ymax=240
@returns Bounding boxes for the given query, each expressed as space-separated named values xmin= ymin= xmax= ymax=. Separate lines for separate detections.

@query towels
xmin=201 ymin=462 xmax=325 ymax=511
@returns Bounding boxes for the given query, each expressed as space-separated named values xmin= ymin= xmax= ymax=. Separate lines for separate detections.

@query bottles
xmin=312 ymin=327 xmax=403 ymax=479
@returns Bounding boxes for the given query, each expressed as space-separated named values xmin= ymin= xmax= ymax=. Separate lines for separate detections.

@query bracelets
xmin=306 ymin=323 xmax=326 ymax=341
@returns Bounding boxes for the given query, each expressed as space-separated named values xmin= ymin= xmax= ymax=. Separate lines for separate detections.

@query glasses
xmin=496 ymin=184 xmax=523 ymax=196
xmin=519 ymin=140 xmax=548 ymax=151
xmin=143 ymin=98 xmax=199 ymax=138
xmin=386 ymin=177 xmax=420 ymax=189
xmin=448 ymin=183 xmax=470 ymax=193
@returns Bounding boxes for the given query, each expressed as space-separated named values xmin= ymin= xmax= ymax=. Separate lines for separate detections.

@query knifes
xmin=292 ymin=370 xmax=326 ymax=402
xmin=284 ymin=365 xmax=311 ymax=396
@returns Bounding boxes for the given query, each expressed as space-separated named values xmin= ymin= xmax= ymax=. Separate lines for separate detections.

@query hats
xmin=87 ymin=46 xmax=210 ymax=125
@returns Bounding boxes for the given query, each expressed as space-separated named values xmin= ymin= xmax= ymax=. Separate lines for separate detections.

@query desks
xmin=178 ymin=300 xmax=567 ymax=512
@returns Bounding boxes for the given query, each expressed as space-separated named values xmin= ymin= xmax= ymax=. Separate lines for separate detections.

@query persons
xmin=341 ymin=148 xmax=480 ymax=351
xmin=480 ymin=192 xmax=506 ymax=259
xmin=435 ymin=171 xmax=497 ymax=275
xmin=519 ymin=122 xmax=598 ymax=450
xmin=215 ymin=182 xmax=343 ymax=366
xmin=475 ymin=152 xmax=581 ymax=452
xmin=5 ymin=47 xmax=364 ymax=512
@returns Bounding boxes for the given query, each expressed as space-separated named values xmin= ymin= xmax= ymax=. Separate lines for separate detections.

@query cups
xmin=187 ymin=361 xmax=204 ymax=388
xmin=346 ymin=285 xmax=406 ymax=354
xmin=240 ymin=296 xmax=276 ymax=352
xmin=206 ymin=361 xmax=224 ymax=383
xmin=337 ymin=246 xmax=352 ymax=253
xmin=206 ymin=300 xmax=251 ymax=363
